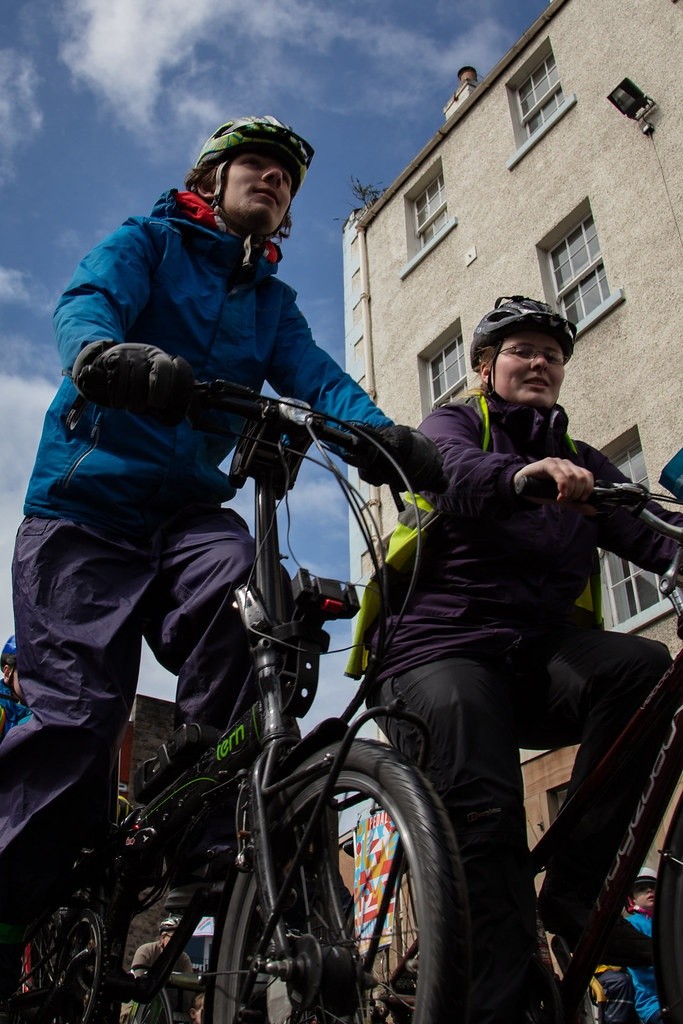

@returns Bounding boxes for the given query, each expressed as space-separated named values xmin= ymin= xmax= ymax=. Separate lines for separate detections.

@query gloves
xmin=71 ymin=339 xmax=195 ymax=427
xmin=360 ymin=425 xmax=443 ymax=494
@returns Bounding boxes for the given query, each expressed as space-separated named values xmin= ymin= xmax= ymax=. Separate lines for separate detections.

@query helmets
xmin=159 ymin=918 xmax=182 ymax=936
xmin=194 ymin=115 xmax=315 ymax=197
xmin=634 ymin=866 xmax=657 ymax=882
xmin=469 ymin=296 xmax=578 ymax=372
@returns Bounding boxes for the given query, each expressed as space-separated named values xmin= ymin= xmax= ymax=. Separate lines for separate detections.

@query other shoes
xmin=538 ymin=892 xmax=656 ymax=966
xmin=163 ymin=862 xmax=298 ymax=915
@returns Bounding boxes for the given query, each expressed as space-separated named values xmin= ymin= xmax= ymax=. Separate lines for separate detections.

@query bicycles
xmin=0 ymin=359 xmax=473 ymax=1024
xmin=362 ymin=473 xmax=683 ymax=1024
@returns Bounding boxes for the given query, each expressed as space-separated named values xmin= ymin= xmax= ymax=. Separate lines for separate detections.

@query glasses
xmin=498 ymin=344 xmax=569 ymax=365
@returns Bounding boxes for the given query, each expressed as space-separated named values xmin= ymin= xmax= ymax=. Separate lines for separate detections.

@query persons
xmin=189 ymin=992 xmax=206 ymax=1024
xmin=0 ymin=115 xmax=447 ymax=1024
xmin=594 ymin=865 xmax=663 ymax=1024
xmin=130 ymin=917 xmax=198 ymax=1024
xmin=364 ymin=294 xmax=682 ymax=1024
xmin=0 ymin=636 xmax=35 ymax=747
